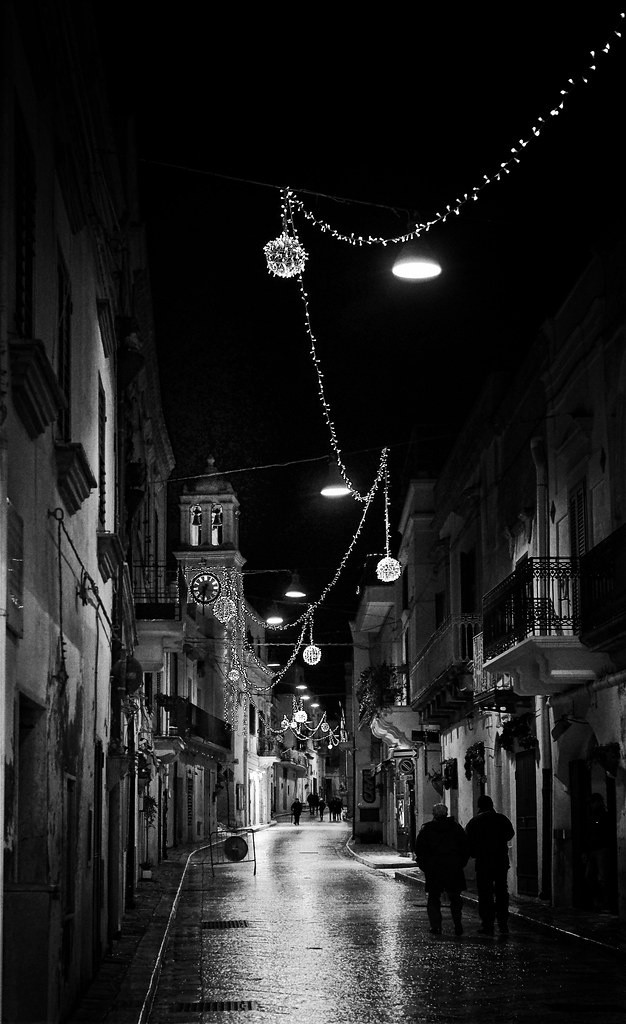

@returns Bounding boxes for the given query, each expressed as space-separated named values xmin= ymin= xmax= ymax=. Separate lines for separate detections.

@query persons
xmin=291 ymin=791 xmax=343 ymax=825
xmin=464 ymin=796 xmax=515 ymax=934
xmin=413 ymin=804 xmax=470 ymax=934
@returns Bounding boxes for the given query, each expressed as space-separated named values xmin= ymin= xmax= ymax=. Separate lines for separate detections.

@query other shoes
xmin=455 ymin=922 xmax=463 ymax=936
xmin=430 ymin=926 xmax=442 ymax=934
xmin=500 ymin=926 xmax=509 ymax=934
xmin=477 ymin=929 xmax=494 ymax=936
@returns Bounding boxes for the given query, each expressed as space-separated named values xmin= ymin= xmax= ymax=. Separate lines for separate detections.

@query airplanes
xmin=128 ymin=453 xmax=351 ymax=500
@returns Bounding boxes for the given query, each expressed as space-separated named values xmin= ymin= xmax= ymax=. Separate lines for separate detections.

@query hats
xmin=477 ymin=795 xmax=494 ymax=809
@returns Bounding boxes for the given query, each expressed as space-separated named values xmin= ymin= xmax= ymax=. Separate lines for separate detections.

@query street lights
xmin=266 ymin=600 xmax=394 ymax=626
xmin=146 ymin=569 xmax=306 ymax=598
xmin=100 ymin=151 xmax=443 ymax=284
